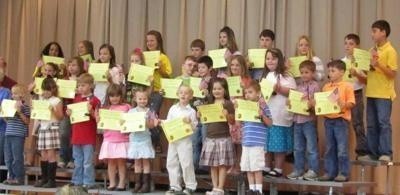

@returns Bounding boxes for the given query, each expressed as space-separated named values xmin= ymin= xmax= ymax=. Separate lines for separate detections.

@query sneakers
xmin=11 ymin=180 xmax=24 ymax=184
xmin=319 ymin=175 xmax=334 ymax=181
xmin=335 ymin=175 xmax=348 ymax=181
xmin=183 ymin=188 xmax=192 ymax=195
xmin=206 ymin=187 xmax=216 ymax=195
xmin=166 ymin=189 xmax=183 ymax=195
xmin=254 ymin=189 xmax=263 ymax=195
xmin=286 ymin=171 xmax=304 ymax=180
xmin=304 ymin=170 xmax=319 ymax=181
xmin=3 ymin=179 xmax=10 ymax=184
xmin=246 ymin=189 xmax=254 ymax=195
xmin=214 ymin=189 xmax=224 ymax=195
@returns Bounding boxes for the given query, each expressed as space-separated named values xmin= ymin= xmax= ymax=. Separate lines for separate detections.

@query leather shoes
xmin=379 ymin=155 xmax=392 ymax=161
xmin=115 ymin=187 xmax=126 ymax=191
xmin=107 ymin=184 xmax=116 ymax=190
xmin=357 ymin=154 xmax=376 ymax=160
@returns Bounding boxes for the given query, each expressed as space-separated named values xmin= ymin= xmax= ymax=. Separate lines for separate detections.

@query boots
xmin=138 ymin=173 xmax=150 ymax=193
xmin=34 ymin=160 xmax=48 ymax=187
xmin=131 ymin=172 xmax=143 ymax=193
xmin=41 ymin=161 xmax=57 ymax=187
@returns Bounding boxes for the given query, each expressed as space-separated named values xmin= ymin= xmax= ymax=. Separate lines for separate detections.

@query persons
xmin=64 ymin=73 xmax=101 ymax=192
xmin=87 ymin=45 xmax=125 ymax=168
xmin=233 ymin=80 xmax=273 ymax=195
xmin=336 ymin=34 xmax=369 ymax=161
xmin=0 ymin=84 xmax=30 ymax=186
xmin=156 ymin=86 xmax=198 ymax=195
xmin=94 ymin=84 xmax=132 ymax=192
xmin=122 ymin=49 xmax=155 ymax=112
xmin=57 ymin=57 xmax=86 ymax=168
xmin=286 ymin=61 xmax=320 ymax=181
xmin=120 ymin=92 xmax=159 ymax=193
xmin=196 ymin=78 xmax=233 ymax=195
xmin=256 ymin=48 xmax=297 ymax=178
xmin=0 ymin=58 xmax=18 ymax=90
xmin=31 ymin=76 xmax=64 ymax=188
xmin=352 ymin=20 xmax=397 ymax=162
xmin=190 ymin=39 xmax=205 ymax=63
xmin=189 ymin=57 xmax=216 ymax=173
xmin=312 ymin=60 xmax=355 ymax=183
xmin=160 ymin=56 xmax=197 ymax=110
xmin=224 ymin=56 xmax=253 ymax=177
xmin=246 ymin=29 xmax=275 ymax=84
xmin=208 ymin=26 xmax=241 ymax=80
xmin=286 ymin=35 xmax=325 ymax=84
xmin=0 ymin=82 xmax=12 ymax=180
xmin=32 ymin=42 xmax=65 ymax=103
xmin=140 ymin=31 xmax=172 ymax=153
xmin=29 ymin=63 xmax=63 ymax=98
xmin=77 ymin=41 xmax=94 ymax=73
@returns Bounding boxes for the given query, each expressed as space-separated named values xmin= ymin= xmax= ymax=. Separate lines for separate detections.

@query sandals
xmin=265 ymin=169 xmax=283 ymax=178
xmin=262 ymin=170 xmax=271 ymax=175
